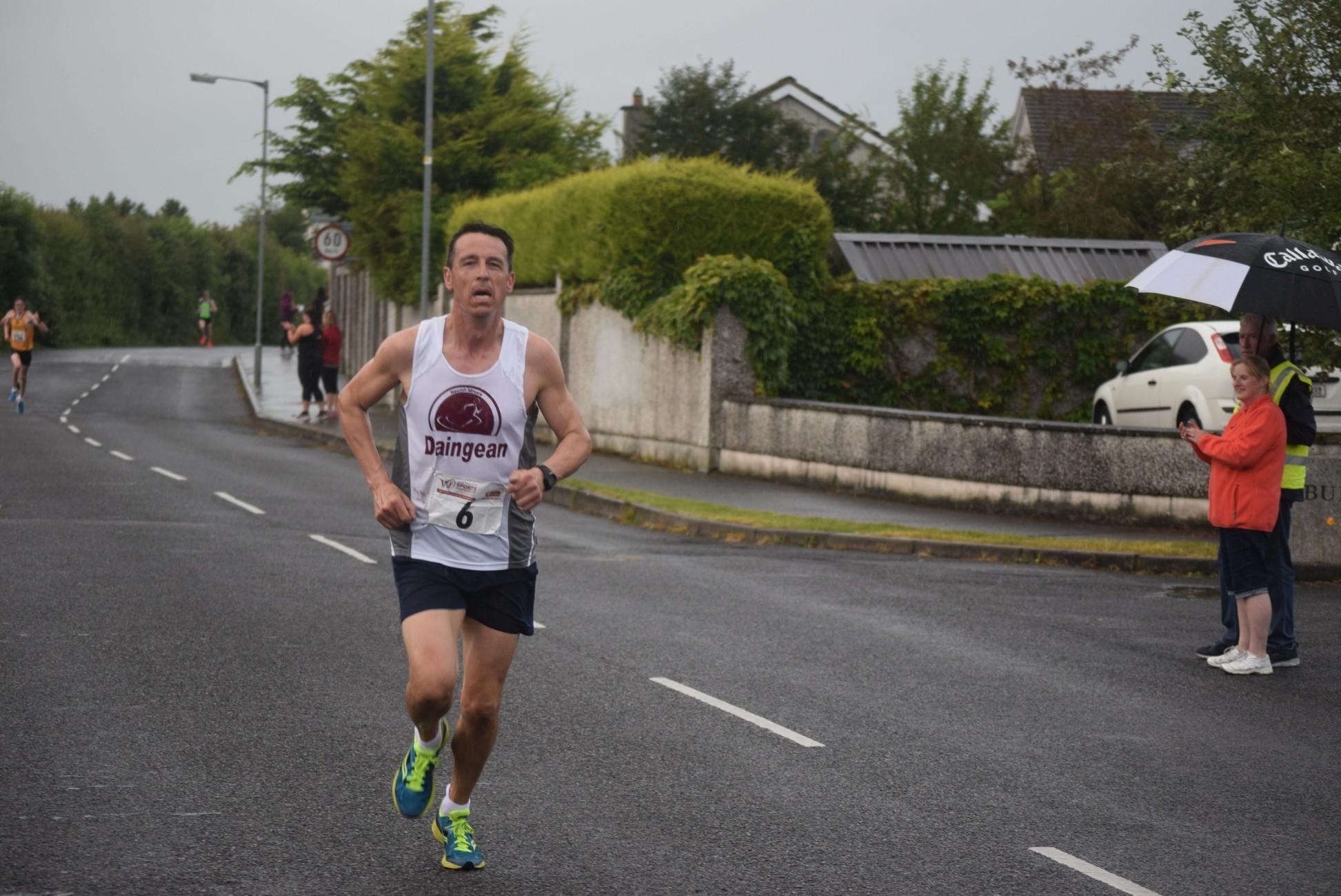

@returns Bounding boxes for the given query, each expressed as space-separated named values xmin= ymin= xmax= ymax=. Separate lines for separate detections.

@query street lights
xmin=189 ymin=72 xmax=268 ymax=396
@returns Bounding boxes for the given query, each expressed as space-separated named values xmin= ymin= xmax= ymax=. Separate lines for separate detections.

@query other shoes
xmin=16 ymin=395 xmax=24 ymax=413
xmin=207 ymin=341 xmax=213 ymax=348
xmin=297 ymin=411 xmax=309 ymax=419
xmin=316 ymin=411 xmax=327 ymax=418
xmin=8 ymin=384 xmax=20 ymax=402
xmin=200 ymin=335 xmax=207 ymax=345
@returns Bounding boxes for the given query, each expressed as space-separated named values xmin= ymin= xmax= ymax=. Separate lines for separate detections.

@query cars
xmin=1089 ymin=319 xmax=1341 ymax=445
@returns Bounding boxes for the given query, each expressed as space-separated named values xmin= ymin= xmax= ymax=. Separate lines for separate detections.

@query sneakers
xmin=1267 ymin=644 xmax=1300 ymax=668
xmin=431 ymin=797 xmax=487 ymax=869
xmin=1206 ymin=645 xmax=1248 ymax=667
xmin=1195 ymin=640 xmax=1230 ymax=659
xmin=392 ymin=714 xmax=449 ymax=819
xmin=1221 ymin=650 xmax=1273 ymax=674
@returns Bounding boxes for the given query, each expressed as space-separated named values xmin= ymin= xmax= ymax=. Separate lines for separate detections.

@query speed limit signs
xmin=314 ymin=225 xmax=352 ymax=262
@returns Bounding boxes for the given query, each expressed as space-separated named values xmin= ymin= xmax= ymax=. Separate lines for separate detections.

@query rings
xmin=1191 ymin=434 xmax=1195 ymax=437
xmin=1181 ymin=436 xmax=1185 ymax=439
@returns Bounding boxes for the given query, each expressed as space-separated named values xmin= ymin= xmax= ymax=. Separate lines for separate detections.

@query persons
xmin=281 ymin=289 xmax=299 ymax=348
xmin=1125 ymin=229 xmax=1341 ymax=358
xmin=335 ymin=221 xmax=594 ymax=870
xmin=1178 ymin=355 xmax=1287 ymax=675
xmin=195 ymin=286 xmax=219 ymax=347
xmin=318 ymin=302 xmax=342 ymax=419
xmin=1194 ymin=311 xmax=1316 ymax=668
xmin=281 ymin=302 xmax=329 ymax=422
xmin=314 ymin=285 xmax=329 ymax=318
xmin=1 ymin=293 xmax=51 ymax=413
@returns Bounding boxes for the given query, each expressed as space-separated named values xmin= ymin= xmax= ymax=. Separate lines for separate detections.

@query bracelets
xmin=531 ymin=463 xmax=558 ymax=493
